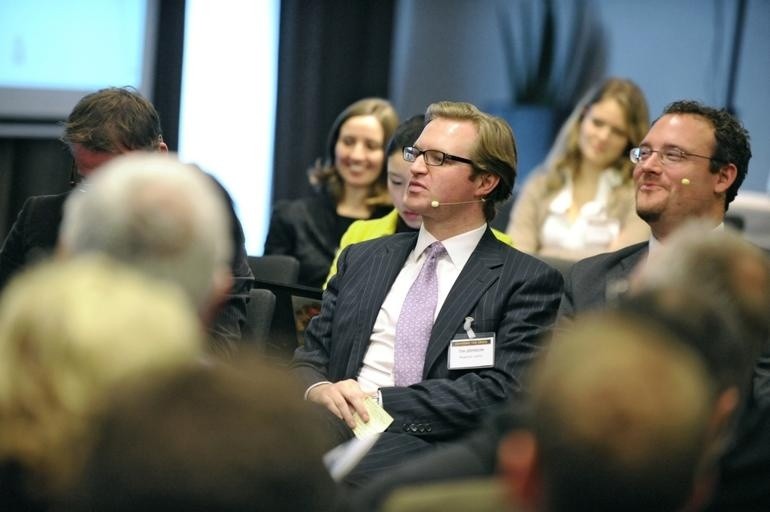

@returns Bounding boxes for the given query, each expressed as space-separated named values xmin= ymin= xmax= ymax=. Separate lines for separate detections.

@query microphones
xmin=430 ymin=198 xmax=486 ymax=207
xmin=680 ymin=178 xmax=726 ymax=199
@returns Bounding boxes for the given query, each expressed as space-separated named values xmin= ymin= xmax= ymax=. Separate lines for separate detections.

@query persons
xmin=0 ymin=85 xmax=356 ymax=512
xmin=499 ymin=75 xmax=770 ymax=509
xmin=265 ymin=100 xmax=563 ymax=510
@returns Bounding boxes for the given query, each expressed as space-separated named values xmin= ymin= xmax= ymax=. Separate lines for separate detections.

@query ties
xmin=394 ymin=242 xmax=446 ymax=386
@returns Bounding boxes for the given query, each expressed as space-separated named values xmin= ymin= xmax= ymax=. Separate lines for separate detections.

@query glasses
xmin=629 ymin=147 xmax=711 ymax=160
xmin=401 ymin=145 xmax=475 ymax=166
